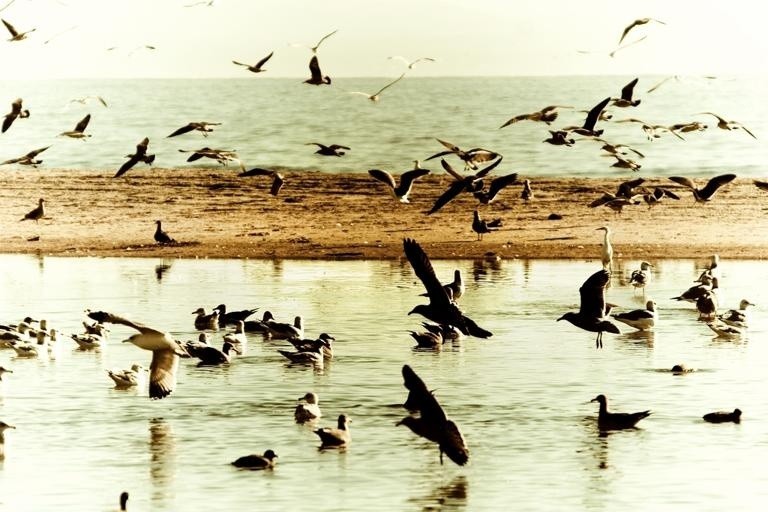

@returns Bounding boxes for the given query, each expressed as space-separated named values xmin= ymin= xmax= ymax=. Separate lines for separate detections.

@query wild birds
xmin=1 ymin=1 xmax=768 ymax=247
xmin=1 ymin=303 xmax=176 ymax=400
xmin=590 ymin=393 xmax=653 ymax=432
xmin=230 ymin=449 xmax=280 ymax=470
xmin=400 ymin=237 xmax=493 ymax=352
xmin=0 ymin=421 xmax=14 ymax=459
xmin=177 ymin=302 xmax=338 ymax=371
xmin=152 ymin=260 xmax=173 ymax=283
xmin=392 ymin=363 xmax=472 ymax=468
xmin=295 ymin=392 xmax=352 ymax=449
xmin=556 ymin=223 xmax=756 ymax=349
